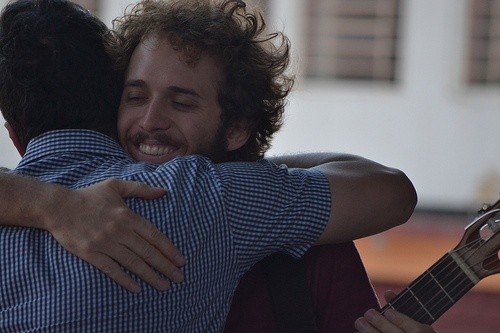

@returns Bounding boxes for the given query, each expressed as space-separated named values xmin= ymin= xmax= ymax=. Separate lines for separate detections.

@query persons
xmin=0 ymin=1 xmax=438 ymax=333
xmin=0 ymin=3 xmax=421 ymax=333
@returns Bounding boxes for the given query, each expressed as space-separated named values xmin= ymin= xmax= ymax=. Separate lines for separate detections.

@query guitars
xmin=368 ymin=201 xmax=499 ymax=332
